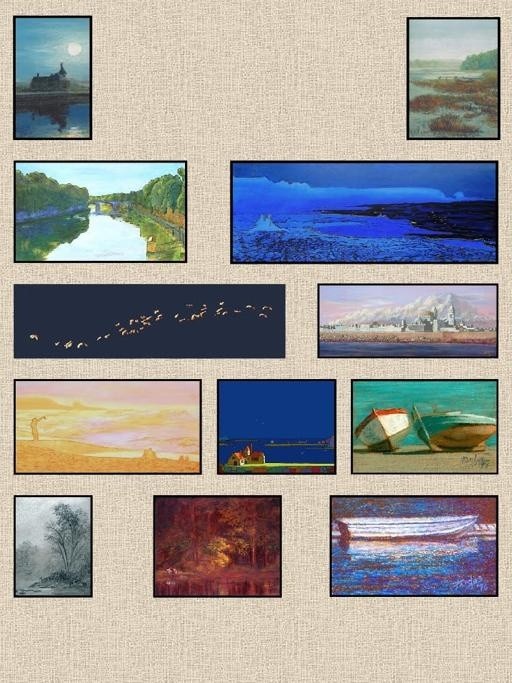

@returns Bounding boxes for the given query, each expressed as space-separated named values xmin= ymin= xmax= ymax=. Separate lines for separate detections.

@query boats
xmin=410 ymin=404 xmax=496 ymax=453
xmin=355 ymin=406 xmax=410 ymax=453
